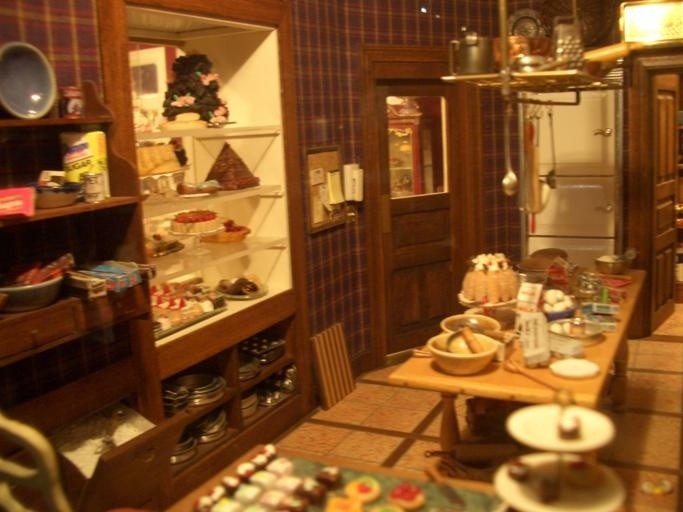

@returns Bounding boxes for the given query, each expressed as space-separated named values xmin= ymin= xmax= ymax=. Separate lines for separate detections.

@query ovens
xmin=516 ymin=86 xmax=625 ymax=265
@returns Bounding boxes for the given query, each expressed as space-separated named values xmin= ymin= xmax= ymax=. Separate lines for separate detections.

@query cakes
xmin=560 ymin=415 xmax=580 ymax=439
xmin=146 ymin=210 xmax=251 ymax=254
xmin=136 ymin=136 xmax=260 ymax=195
xmin=133 ymin=54 xmax=229 ymax=134
xmin=192 ymin=443 xmax=428 ymax=512
xmin=149 ymin=274 xmax=262 ymax=335
xmin=568 ymin=461 xmax=594 ymax=482
xmin=509 ymin=461 xmax=529 ymax=481
xmin=462 ymin=252 xmax=519 ymax=304
xmin=539 ymin=476 xmax=560 ymax=502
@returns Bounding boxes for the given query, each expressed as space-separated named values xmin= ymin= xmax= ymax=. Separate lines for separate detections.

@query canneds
xmin=57 ymin=130 xmax=111 ymax=203
xmin=571 ymin=318 xmax=586 ymax=335
xmin=59 ymin=86 xmax=83 ymax=119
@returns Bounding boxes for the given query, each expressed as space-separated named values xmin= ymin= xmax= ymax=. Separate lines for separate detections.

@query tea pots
xmin=446 ymin=34 xmax=493 ymax=76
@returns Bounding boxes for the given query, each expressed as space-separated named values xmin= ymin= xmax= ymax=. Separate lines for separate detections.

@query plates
xmin=547 ymin=359 xmax=600 ymax=379
xmin=213 ymin=283 xmax=269 ymax=301
xmin=238 ymin=355 xmax=261 ymax=381
xmin=168 ymin=407 xmax=228 ymax=464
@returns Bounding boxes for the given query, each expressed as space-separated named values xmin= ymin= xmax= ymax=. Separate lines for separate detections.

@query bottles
xmin=570 ymin=310 xmax=585 ymax=333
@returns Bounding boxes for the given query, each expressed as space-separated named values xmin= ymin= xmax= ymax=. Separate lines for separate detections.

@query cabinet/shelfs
xmin=0 ymin=0 xmax=318 ymax=511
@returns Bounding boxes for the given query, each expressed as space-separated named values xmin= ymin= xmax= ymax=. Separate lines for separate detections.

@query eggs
xmin=641 ymin=479 xmax=672 ymax=496
xmin=542 ymin=289 xmax=573 ymax=313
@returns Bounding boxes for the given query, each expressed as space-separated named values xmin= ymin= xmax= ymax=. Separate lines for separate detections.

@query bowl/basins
xmin=173 ymin=374 xmax=227 ymax=407
xmin=443 ymin=442 xmax=519 ymax=485
xmin=440 ymin=313 xmax=500 ymax=336
xmin=515 ymin=261 xmax=547 ymax=281
xmin=494 ymin=36 xmax=552 ymax=71
xmin=239 ymin=389 xmax=259 ymax=418
xmin=0 ymin=275 xmax=61 ymax=313
xmin=23 ymin=181 xmax=80 ymax=209
xmin=0 ymin=41 xmax=57 ymax=119
xmin=595 ymin=254 xmax=631 ymax=274
xmin=426 ymin=330 xmax=496 ymax=376
xmin=545 ymin=306 xmax=574 ymax=321
xmin=456 ymin=292 xmax=517 ymax=323
